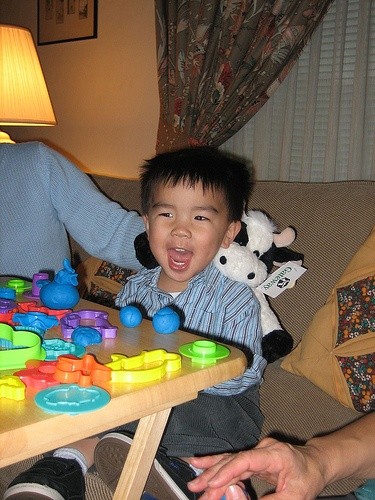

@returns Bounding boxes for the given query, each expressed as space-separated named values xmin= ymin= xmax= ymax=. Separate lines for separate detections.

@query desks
xmin=0 ymin=274 xmax=248 ymax=500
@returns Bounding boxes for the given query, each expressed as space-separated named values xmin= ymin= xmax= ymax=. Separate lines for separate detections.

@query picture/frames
xmin=37 ymin=0 xmax=98 ymax=46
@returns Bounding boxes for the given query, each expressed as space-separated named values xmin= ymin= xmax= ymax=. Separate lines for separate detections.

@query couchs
xmin=0 ymin=172 xmax=375 ymax=500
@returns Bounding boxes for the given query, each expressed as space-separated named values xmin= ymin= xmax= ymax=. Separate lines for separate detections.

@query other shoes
xmin=1 ymin=452 xmax=86 ymax=500
xmin=94 ymin=429 xmax=206 ymax=500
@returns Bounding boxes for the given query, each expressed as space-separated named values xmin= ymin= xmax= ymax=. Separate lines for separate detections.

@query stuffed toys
xmin=133 ymin=210 xmax=303 ymax=365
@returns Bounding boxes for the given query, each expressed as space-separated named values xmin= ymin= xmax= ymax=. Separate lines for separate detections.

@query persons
xmin=0 ymin=141 xmax=147 ymax=286
xmin=4 ymin=147 xmax=269 ymax=500
xmin=188 ymin=409 xmax=375 ymax=500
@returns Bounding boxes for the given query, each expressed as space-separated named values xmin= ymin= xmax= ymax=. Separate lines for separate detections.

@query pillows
xmin=279 ymin=228 xmax=375 ymax=414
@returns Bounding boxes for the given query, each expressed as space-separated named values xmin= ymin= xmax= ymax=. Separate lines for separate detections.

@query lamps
xmin=0 ymin=24 xmax=57 ymax=145
